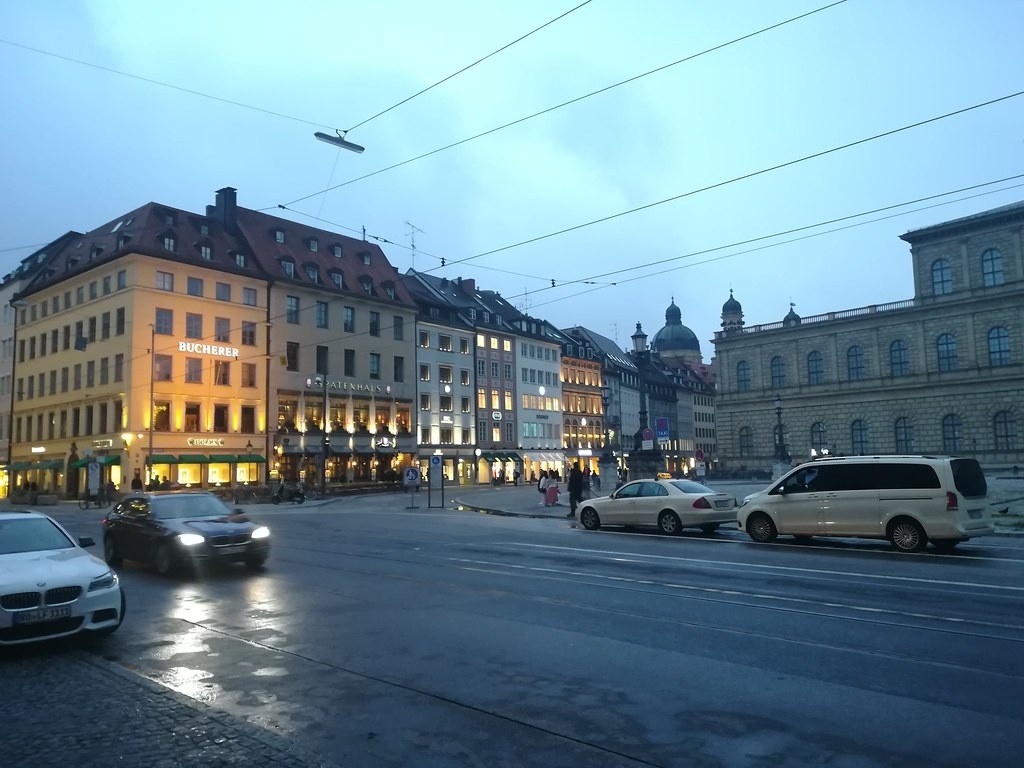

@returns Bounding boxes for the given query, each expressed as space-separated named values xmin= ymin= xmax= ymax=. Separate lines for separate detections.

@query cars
xmin=102 ymin=489 xmax=270 ymax=577
xmin=658 ymin=473 xmax=672 ymax=479
xmin=0 ymin=507 xmax=125 ymax=647
xmin=575 ymin=479 xmax=739 ymax=536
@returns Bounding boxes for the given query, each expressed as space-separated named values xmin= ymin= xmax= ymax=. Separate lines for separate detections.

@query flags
xmin=74 ymin=336 xmax=88 ymax=352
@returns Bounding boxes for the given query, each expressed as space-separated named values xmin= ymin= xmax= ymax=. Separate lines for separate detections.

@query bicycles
xmin=78 ymin=486 xmax=111 ymax=509
xmin=296 ymin=482 xmax=318 ymax=501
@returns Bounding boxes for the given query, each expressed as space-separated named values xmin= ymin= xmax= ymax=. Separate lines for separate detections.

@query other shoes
xmin=567 ymin=513 xmax=575 ymax=517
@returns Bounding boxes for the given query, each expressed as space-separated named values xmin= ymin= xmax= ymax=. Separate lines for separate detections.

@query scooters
xmin=272 ymin=484 xmax=305 ymax=505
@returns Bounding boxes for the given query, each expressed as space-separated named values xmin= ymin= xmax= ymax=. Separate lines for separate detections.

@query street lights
xmin=624 ymin=321 xmax=667 ymax=478
xmin=771 ymin=394 xmax=793 ymax=480
xmin=597 ymin=384 xmax=619 ymax=489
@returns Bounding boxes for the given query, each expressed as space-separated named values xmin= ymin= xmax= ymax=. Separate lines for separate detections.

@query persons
xmin=582 ymin=465 xmax=591 ymax=479
xmin=591 ymin=470 xmax=598 ymax=486
xmin=384 ymin=467 xmax=429 ymax=493
xmin=513 ymin=469 xmax=519 ymax=486
xmin=538 ymin=468 xmax=561 ymax=507
xmin=131 ymin=474 xmax=171 ymax=492
xmin=617 ymin=467 xmax=628 ymax=482
xmin=23 ymin=479 xmax=37 ymax=492
xmin=566 ymin=462 xmax=584 ymax=516
xmin=498 ymin=469 xmax=505 ymax=480
xmin=530 ymin=472 xmax=536 ymax=485
xmin=107 ymin=478 xmax=116 ymax=505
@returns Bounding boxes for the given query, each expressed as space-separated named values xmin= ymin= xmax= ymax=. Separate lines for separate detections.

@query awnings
xmin=280 ymin=445 xmax=416 ymax=453
xmin=71 ymin=455 xmax=121 ymax=468
xmin=0 ymin=460 xmax=64 ymax=470
xmin=525 ymin=452 xmax=569 ymax=461
xmin=482 ymin=453 xmax=524 ymax=463
xmin=145 ymin=454 xmax=265 ymax=464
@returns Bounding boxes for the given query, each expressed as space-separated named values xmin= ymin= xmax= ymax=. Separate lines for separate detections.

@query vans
xmin=736 ymin=455 xmax=994 ymax=553
